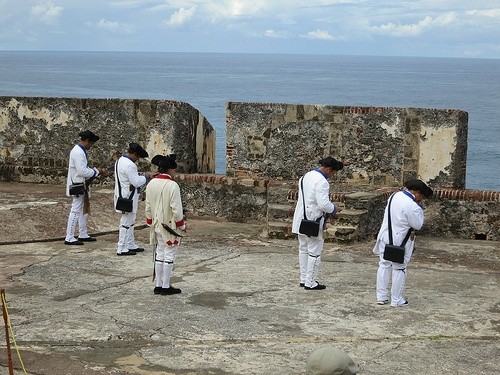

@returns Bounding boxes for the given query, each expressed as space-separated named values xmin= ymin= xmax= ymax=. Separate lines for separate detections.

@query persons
xmin=64 ymin=131 xmax=106 ymax=245
xmin=292 ymin=156 xmax=343 ymax=290
xmin=114 ymin=143 xmax=153 ymax=255
xmin=146 ymin=154 xmax=186 ymax=295
xmin=373 ymin=179 xmax=433 ymax=307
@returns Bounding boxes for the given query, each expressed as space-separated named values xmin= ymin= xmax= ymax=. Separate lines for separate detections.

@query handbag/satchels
xmin=383 ymin=244 xmax=406 ymax=264
xmin=115 ymin=197 xmax=134 ymax=215
xmin=69 ymin=182 xmax=85 ymax=198
xmin=298 ymin=218 xmax=319 ymax=237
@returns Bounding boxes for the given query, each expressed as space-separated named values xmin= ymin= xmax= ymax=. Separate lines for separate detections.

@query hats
xmin=404 ymin=178 xmax=433 ymax=198
xmin=79 ymin=130 xmax=100 ymax=142
xmin=129 ymin=142 xmax=148 ymax=159
xmin=319 ymin=156 xmax=343 ymax=172
xmin=151 ymin=155 xmax=177 ymax=168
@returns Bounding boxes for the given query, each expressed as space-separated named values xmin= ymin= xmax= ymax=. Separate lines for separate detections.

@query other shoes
xmin=305 ymin=282 xmax=326 ymax=290
xmin=128 ymin=247 xmax=145 ymax=252
xmin=154 ymin=286 xmax=162 ymax=294
xmin=301 ymin=281 xmax=318 ymax=288
xmin=116 ymin=251 xmax=136 ymax=255
xmin=162 ymin=285 xmax=181 ymax=294
xmin=378 ymin=299 xmax=388 ymax=304
xmin=78 ymin=236 xmax=97 ymax=242
xmin=64 ymin=240 xmax=85 ymax=245
